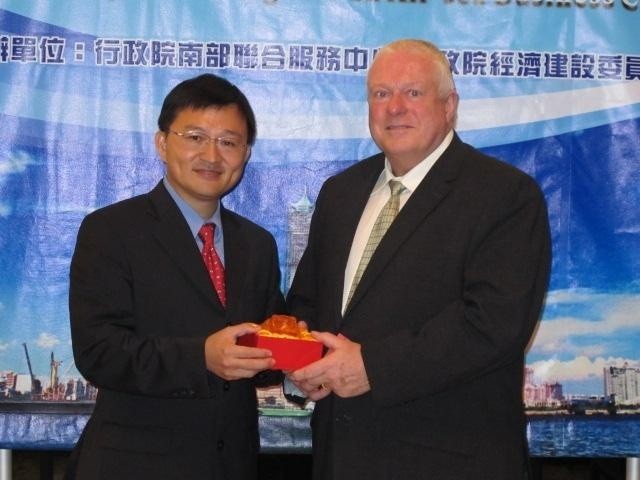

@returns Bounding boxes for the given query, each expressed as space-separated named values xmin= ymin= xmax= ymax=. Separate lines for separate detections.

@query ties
xmin=196 ymin=222 xmax=226 ymax=307
xmin=345 ymin=181 xmax=406 ymax=307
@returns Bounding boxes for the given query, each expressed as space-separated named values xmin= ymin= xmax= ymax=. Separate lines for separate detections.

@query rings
xmin=321 ymin=384 xmax=327 ymax=390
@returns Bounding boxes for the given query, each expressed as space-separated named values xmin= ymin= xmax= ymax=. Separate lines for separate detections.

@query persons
xmin=283 ymin=39 xmax=552 ymax=479
xmin=68 ymin=74 xmax=307 ymax=479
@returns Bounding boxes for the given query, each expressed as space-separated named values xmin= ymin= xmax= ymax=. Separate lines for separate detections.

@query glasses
xmin=174 ymin=129 xmax=245 ymax=153
